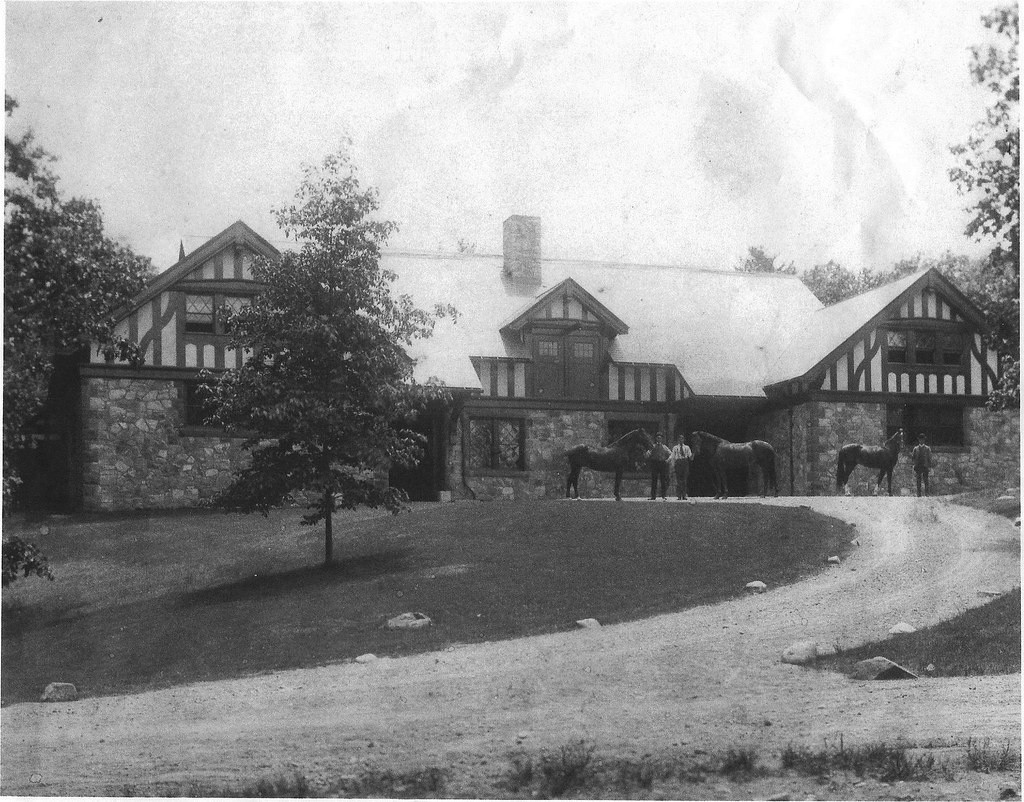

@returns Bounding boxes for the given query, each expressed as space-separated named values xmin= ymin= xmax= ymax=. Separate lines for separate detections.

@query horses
xmin=563 ymin=428 xmax=654 ymax=501
xmin=835 ymin=428 xmax=906 ymax=497
xmin=692 ymin=431 xmax=779 ymax=499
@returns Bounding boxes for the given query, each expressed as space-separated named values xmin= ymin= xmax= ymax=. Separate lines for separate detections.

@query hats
xmin=654 ymin=432 xmax=663 ymax=437
xmin=917 ymin=433 xmax=927 ymax=439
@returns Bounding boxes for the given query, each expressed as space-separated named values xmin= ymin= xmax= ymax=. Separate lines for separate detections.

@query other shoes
xmin=647 ymin=498 xmax=656 ymax=500
xmin=677 ymin=497 xmax=687 ymax=500
xmin=663 ymin=498 xmax=667 ymax=500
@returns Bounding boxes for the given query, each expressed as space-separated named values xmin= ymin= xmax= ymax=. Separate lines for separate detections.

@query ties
xmin=680 ymin=446 xmax=684 ymax=456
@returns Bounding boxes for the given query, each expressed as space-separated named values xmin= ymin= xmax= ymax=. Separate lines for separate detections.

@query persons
xmin=671 ymin=433 xmax=695 ymax=501
xmin=648 ymin=431 xmax=673 ymax=501
xmin=910 ymin=432 xmax=933 ymax=498
